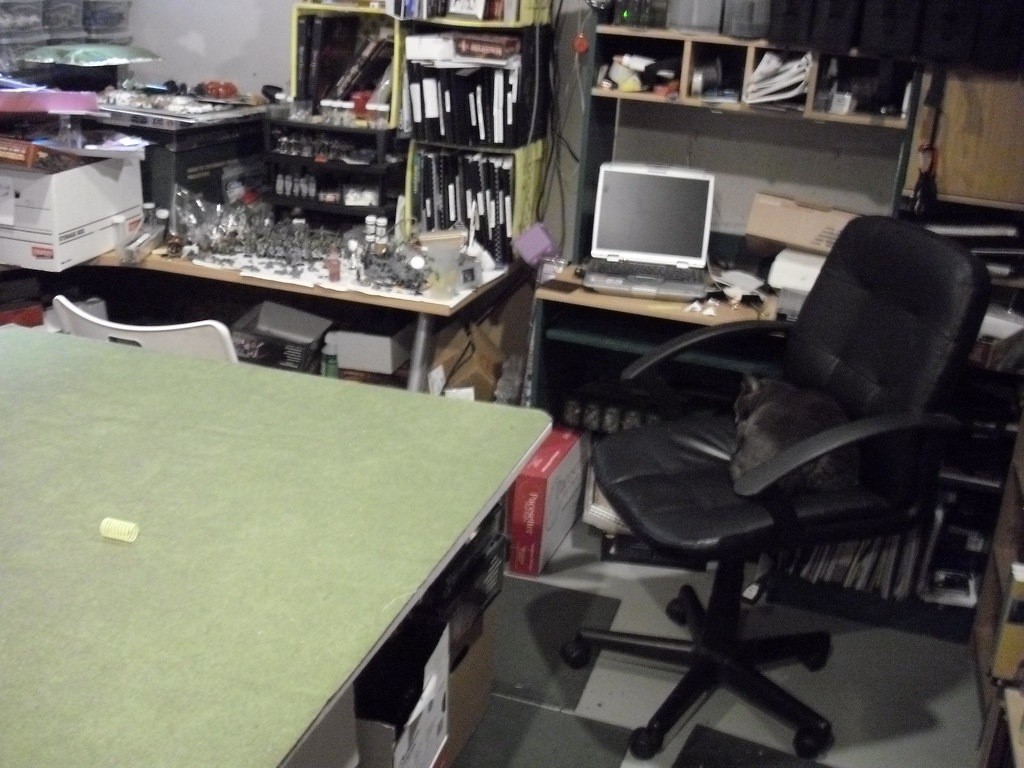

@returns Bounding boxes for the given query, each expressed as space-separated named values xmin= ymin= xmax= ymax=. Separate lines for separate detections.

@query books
xmin=801 ymin=510 xmax=924 ymax=601
xmin=297 ymin=0 xmax=522 ymax=266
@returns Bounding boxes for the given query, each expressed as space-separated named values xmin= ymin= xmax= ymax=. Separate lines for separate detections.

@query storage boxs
xmin=749 ymin=191 xmax=858 ymax=251
xmin=427 ymin=573 xmax=484 ymax=662
xmin=769 ymin=248 xmax=828 ymax=318
xmin=437 ymin=544 xmax=475 ymax=598
xmin=471 ymin=538 xmax=508 ymax=602
xmin=225 ymin=297 xmax=324 ymax=370
xmin=404 ymin=317 xmax=468 ymax=366
xmin=0 ymin=0 xmax=134 ymax=75
xmin=512 ymin=427 xmax=590 ymax=573
xmin=0 ymin=156 xmax=146 ymax=277
xmin=426 ymin=320 xmax=508 ymax=399
xmin=96 ymin=110 xmax=270 ymax=213
xmin=347 ymin=614 xmax=449 ymax=768
xmin=260 ymin=294 xmax=333 ymax=341
xmin=473 ymin=499 xmax=506 ymax=551
xmin=327 ymin=318 xmax=416 ymax=375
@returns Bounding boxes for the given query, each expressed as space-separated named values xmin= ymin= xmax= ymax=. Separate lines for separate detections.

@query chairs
xmin=564 ymin=214 xmax=997 ymax=757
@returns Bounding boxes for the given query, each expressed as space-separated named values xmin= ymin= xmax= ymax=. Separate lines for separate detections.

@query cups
xmin=665 ymin=0 xmax=723 ymax=36
xmin=610 ymin=0 xmax=667 ymax=30
xmin=59 ymin=115 xmax=82 ymax=149
xmin=724 ymin=0 xmax=772 ymax=37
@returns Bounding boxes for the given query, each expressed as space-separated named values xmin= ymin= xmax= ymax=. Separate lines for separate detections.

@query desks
xmin=0 ymin=321 xmax=555 ymax=768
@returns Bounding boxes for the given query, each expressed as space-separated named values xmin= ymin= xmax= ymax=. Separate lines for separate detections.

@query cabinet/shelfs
xmin=526 ymin=285 xmax=1024 ymax=648
xmin=591 ymin=22 xmax=918 ymax=128
xmin=292 ymin=0 xmax=553 ymax=257
xmin=968 ymin=408 xmax=1024 ymax=725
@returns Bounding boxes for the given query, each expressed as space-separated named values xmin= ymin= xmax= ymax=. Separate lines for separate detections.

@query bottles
xmin=320 ymin=330 xmax=339 ymax=379
xmin=156 ymin=209 xmax=170 ymax=227
xmin=275 ymin=93 xmax=312 ymax=123
xmin=364 ymin=215 xmax=388 ymax=258
xmin=275 ymin=174 xmax=317 ymax=198
xmin=143 ymin=203 xmax=156 ymax=223
xmin=365 ymin=104 xmax=389 ymax=128
xmin=320 ymin=100 xmax=357 ymax=128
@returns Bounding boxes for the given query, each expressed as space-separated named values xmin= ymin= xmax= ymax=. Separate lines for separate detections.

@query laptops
xmin=583 ymin=162 xmax=715 ymax=302
